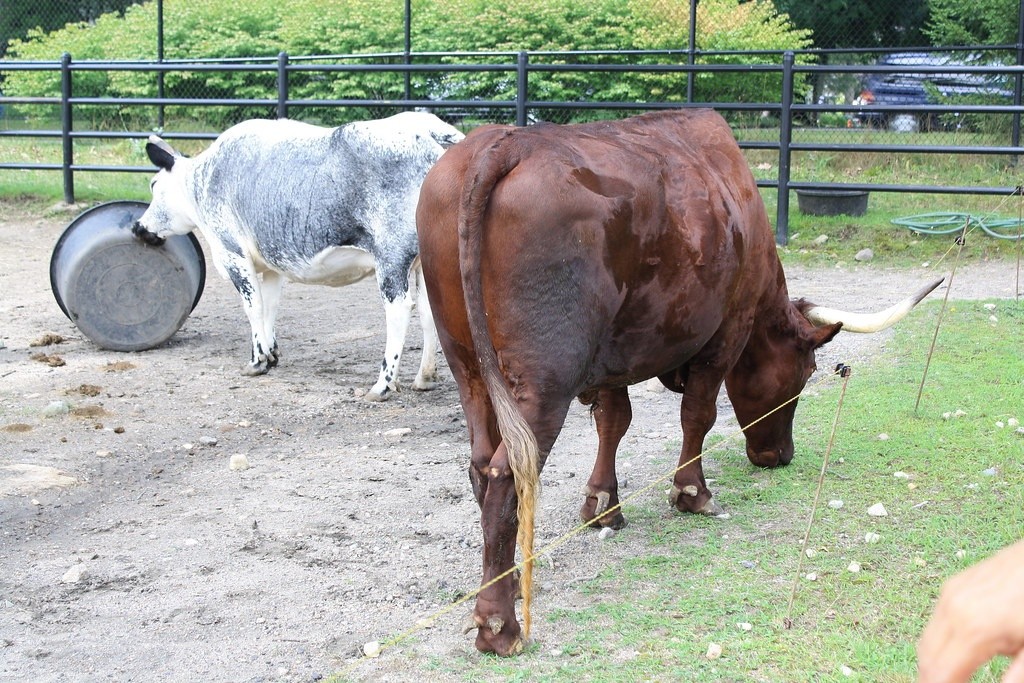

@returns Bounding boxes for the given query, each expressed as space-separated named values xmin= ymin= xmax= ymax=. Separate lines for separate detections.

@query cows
xmin=132 ymin=111 xmax=467 ymax=402
xmin=416 ymin=106 xmax=946 ymax=659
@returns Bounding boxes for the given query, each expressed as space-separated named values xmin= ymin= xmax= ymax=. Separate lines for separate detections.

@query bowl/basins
xmin=794 ymin=188 xmax=869 ymax=218
xmin=50 ymin=200 xmax=206 ymax=353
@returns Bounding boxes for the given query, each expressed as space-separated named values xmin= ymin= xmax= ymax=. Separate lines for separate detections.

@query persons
xmin=916 ymin=537 xmax=1024 ymax=683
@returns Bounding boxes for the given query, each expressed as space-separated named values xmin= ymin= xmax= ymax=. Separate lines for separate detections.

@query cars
xmin=853 ymin=51 xmax=1024 ymax=133
